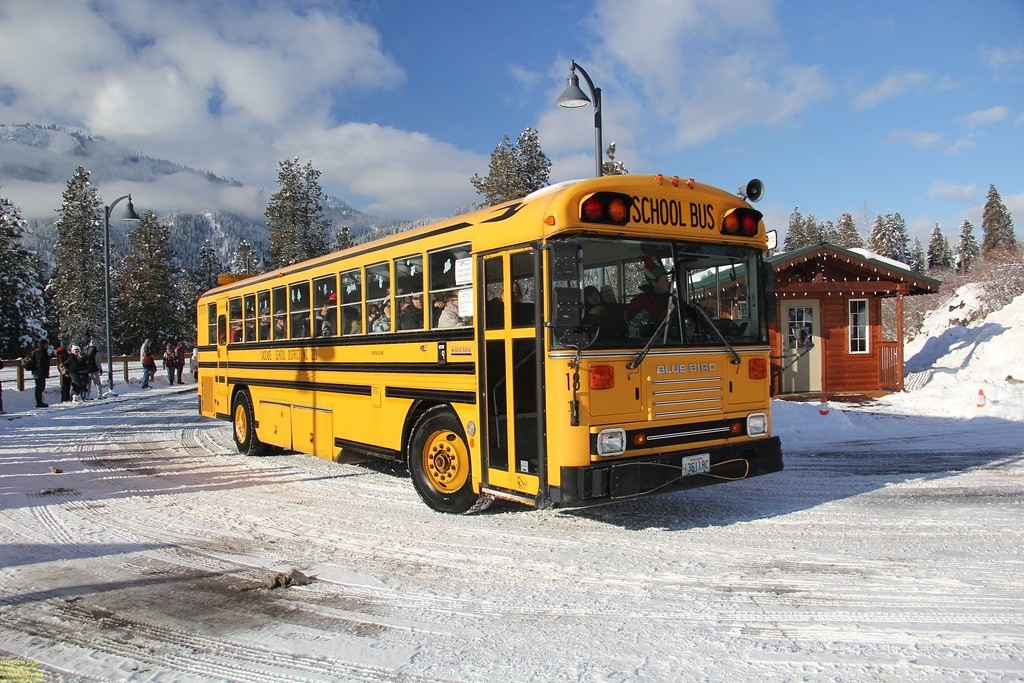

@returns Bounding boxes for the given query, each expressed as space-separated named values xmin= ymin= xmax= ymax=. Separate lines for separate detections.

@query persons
xmin=396 ymin=284 xmax=444 ymax=332
xmin=302 ymin=302 xmax=329 ymax=338
xmin=0 ymin=360 xmax=7 ymax=415
xmin=584 ymin=285 xmax=600 ymax=307
xmin=174 ymin=340 xmax=185 ymax=384
xmin=218 ymin=307 xmax=287 ymax=343
xmin=628 ymin=266 xmax=682 ymax=339
xmin=163 ymin=343 xmax=177 ymax=385
xmin=438 ymin=284 xmax=473 ymax=328
xmin=351 ymin=293 xmax=391 ymax=335
xmin=190 ymin=348 xmax=198 ymax=383
xmin=321 ymin=293 xmax=350 ymax=336
xmin=500 ymin=281 xmax=521 ymax=303
xmin=56 ymin=339 xmax=107 ymax=403
xmin=601 ymin=285 xmax=617 ymax=303
xmin=140 ymin=339 xmax=157 ymax=389
xmin=31 ymin=339 xmax=50 ymax=408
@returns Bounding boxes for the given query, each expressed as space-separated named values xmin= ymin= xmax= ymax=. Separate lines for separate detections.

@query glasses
xmin=263 ymin=313 xmax=270 ymax=316
xmin=277 ymin=317 xmax=286 ymax=320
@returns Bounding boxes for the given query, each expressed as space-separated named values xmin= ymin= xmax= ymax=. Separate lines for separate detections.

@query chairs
xmin=260 ymin=302 xmax=718 ymax=347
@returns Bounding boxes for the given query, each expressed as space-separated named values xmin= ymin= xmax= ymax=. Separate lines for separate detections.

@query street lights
xmin=556 ymin=59 xmax=602 ymax=177
xmin=102 ymin=193 xmax=140 ymax=397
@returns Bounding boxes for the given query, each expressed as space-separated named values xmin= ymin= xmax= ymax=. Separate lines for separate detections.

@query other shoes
xmin=170 ymin=381 xmax=173 ymax=385
xmin=142 ymin=384 xmax=151 ymax=389
xmin=37 ymin=402 xmax=48 ymax=407
xmin=179 ymin=380 xmax=185 ymax=384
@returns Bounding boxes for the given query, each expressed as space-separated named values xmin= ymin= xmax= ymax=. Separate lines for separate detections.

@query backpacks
xmin=24 ymin=348 xmax=41 ymax=371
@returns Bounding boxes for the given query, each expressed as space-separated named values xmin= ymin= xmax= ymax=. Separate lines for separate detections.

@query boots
xmin=73 ymin=394 xmax=83 ymax=402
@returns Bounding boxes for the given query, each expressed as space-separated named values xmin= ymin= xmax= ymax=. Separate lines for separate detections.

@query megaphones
xmin=739 ymin=179 xmax=765 ymax=202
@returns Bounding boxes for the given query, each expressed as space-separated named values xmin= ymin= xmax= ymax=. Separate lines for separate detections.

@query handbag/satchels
xmin=143 ymin=352 xmax=152 ymax=367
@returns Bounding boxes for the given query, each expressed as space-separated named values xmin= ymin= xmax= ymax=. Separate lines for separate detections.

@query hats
xmin=167 ymin=343 xmax=172 ymax=348
xmin=262 ymin=307 xmax=271 ymax=314
xmin=70 ymin=344 xmax=80 ymax=352
xmin=645 ymin=266 xmax=671 ymax=281
xmin=38 ymin=338 xmax=47 ymax=346
xmin=90 ymin=340 xmax=98 ymax=347
xmin=329 ymin=292 xmax=338 ymax=304
xmin=178 ymin=340 xmax=184 ymax=346
xmin=61 ymin=341 xmax=68 ymax=348
xmin=276 ymin=309 xmax=287 ymax=315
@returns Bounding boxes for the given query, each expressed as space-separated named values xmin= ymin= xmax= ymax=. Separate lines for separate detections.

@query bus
xmin=197 ymin=173 xmax=814 ymax=516
